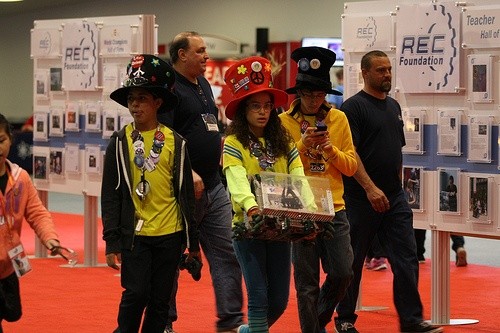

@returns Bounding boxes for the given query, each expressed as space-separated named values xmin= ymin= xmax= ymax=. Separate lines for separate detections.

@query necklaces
xmin=300 ymin=107 xmax=323 ymax=162
xmin=247 ymin=133 xmax=276 ymax=175
xmin=129 ymin=120 xmax=165 ymax=199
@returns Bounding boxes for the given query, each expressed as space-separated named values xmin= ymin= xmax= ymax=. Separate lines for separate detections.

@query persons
xmin=405 ymin=168 xmax=420 ymax=207
xmin=0 ymin=114 xmax=63 ymax=333
xmin=329 ymin=70 xmax=344 ymax=111
xmin=279 ymin=46 xmax=360 ymax=333
xmin=363 ymin=239 xmax=389 ymax=271
xmin=219 ymin=56 xmax=319 ymax=333
xmin=338 ymin=49 xmax=444 ymax=333
xmin=413 ymin=227 xmax=468 ymax=268
xmin=99 ymin=54 xmax=203 ymax=333
xmin=446 ymin=174 xmax=458 ymax=212
xmin=157 ymin=30 xmax=246 ymax=332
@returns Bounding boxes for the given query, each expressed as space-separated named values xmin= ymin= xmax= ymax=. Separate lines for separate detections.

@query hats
xmin=224 ymin=55 xmax=288 ymax=120
xmin=110 ymin=53 xmax=180 ymax=115
xmin=285 ymin=47 xmax=343 ymax=96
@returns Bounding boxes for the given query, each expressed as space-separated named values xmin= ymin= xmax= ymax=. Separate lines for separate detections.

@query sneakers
xmin=400 ymin=319 xmax=445 ymax=333
xmin=365 ymin=257 xmax=387 ymax=270
xmin=456 ymin=246 xmax=467 ymax=266
xmin=417 ymin=253 xmax=426 ymax=264
xmin=334 ymin=314 xmax=359 ymax=333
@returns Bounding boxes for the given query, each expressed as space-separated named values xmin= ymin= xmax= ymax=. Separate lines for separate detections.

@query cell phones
xmin=313 ymin=124 xmax=328 ymax=136
